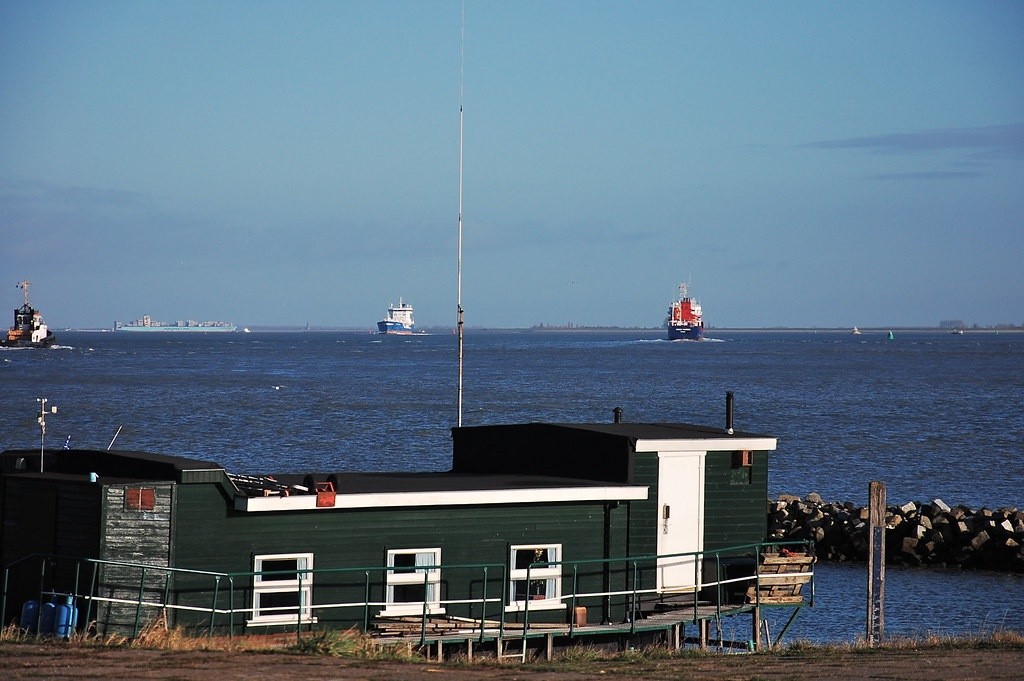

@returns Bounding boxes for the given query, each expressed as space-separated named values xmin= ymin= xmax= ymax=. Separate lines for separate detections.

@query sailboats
xmin=889 ymin=331 xmax=895 ymax=340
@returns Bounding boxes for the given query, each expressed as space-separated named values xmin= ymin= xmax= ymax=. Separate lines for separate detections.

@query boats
xmin=852 ymin=326 xmax=860 ymax=335
xmin=376 ymin=294 xmax=417 ymax=335
xmin=665 ymin=271 xmax=708 ymax=342
xmin=0 ymin=281 xmax=57 ymax=351
xmin=951 ymin=327 xmax=964 ymax=334
